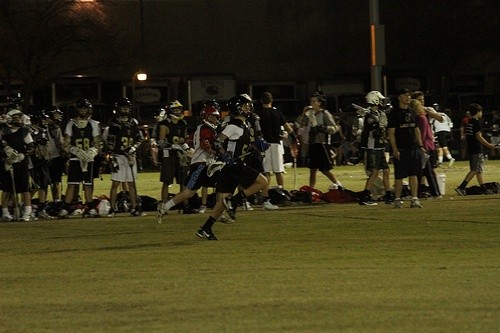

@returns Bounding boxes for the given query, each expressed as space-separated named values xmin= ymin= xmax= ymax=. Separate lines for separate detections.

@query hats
xmin=396 ymin=87 xmax=413 ymax=97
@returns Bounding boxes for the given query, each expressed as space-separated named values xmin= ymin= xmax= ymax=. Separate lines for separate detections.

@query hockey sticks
xmin=4 ymin=158 xmax=20 ymax=222
xmin=290 ymin=144 xmax=299 ymax=188
xmin=128 ymin=152 xmax=141 ymax=216
xmin=480 ymin=140 xmax=500 ymax=159
xmin=80 ymin=158 xmax=88 ymax=218
xmin=207 ymin=151 xmax=252 ymax=178
xmin=303 ymin=106 xmax=335 ymax=167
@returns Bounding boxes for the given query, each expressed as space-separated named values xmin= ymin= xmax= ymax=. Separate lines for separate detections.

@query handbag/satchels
xmin=70 ymin=146 xmax=98 ymax=164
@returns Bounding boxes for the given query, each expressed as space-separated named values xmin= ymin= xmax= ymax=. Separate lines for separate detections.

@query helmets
xmin=0 ymin=91 xmax=93 ymax=129
xmin=152 ymin=98 xmax=184 ymax=126
xmin=226 ymin=92 xmax=255 ymax=119
xmin=365 ymin=90 xmax=387 ymax=107
xmin=112 ymin=96 xmax=133 ymax=120
xmin=198 ymin=99 xmax=222 ymax=131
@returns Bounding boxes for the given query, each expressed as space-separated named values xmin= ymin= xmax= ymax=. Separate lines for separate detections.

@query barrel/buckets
xmin=425 ymin=173 xmax=446 ymax=195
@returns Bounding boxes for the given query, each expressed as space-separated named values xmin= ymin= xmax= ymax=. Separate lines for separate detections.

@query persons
xmin=0 ymin=92 xmax=342 ymax=240
xmin=347 ymin=88 xmax=500 ymax=208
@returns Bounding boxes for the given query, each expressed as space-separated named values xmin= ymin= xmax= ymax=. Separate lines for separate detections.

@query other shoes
xmin=0 ymin=205 xmax=99 ymax=223
xmin=196 ymin=226 xmax=217 ymax=241
xmin=384 ymin=196 xmax=395 ymax=204
xmin=107 ymin=207 xmax=117 ymax=218
xmin=242 ymin=200 xmax=255 ymax=211
xmin=128 ymin=209 xmax=147 ymax=217
xmin=179 ymin=195 xmax=236 ymax=224
xmin=261 ymin=199 xmax=280 ymax=210
xmin=359 ymin=196 xmax=379 ymax=206
xmin=156 ymin=202 xmax=169 ymax=225
xmin=455 ymin=187 xmax=466 ymax=197
xmin=436 ymin=157 xmax=456 ymax=168
xmin=393 ymin=199 xmax=423 ymax=209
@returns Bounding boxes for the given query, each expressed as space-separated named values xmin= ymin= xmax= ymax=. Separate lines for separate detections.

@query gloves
xmin=122 ymin=144 xmax=137 ymax=158
xmin=107 ymin=156 xmax=120 ymax=174
xmin=3 ymin=145 xmax=25 ymax=166
